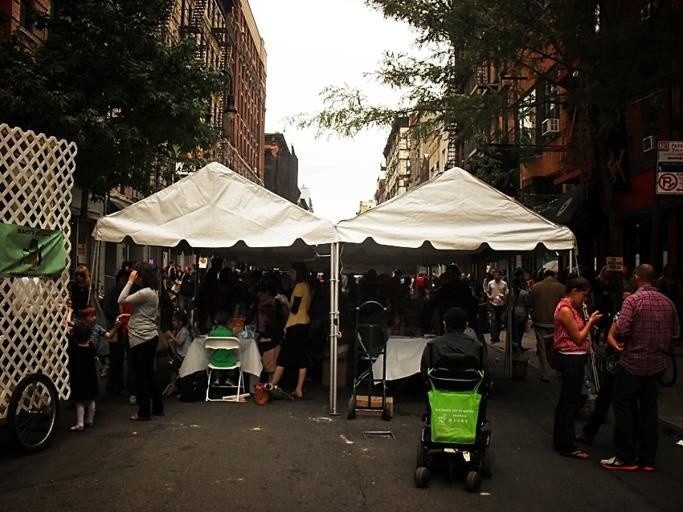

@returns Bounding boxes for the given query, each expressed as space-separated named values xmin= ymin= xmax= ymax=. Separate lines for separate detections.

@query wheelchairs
xmin=413 ymin=363 xmax=493 ymax=490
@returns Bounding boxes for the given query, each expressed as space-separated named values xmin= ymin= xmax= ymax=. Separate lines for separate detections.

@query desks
xmin=371 ymin=335 xmax=440 ymax=386
xmin=178 ymin=326 xmax=264 ymax=380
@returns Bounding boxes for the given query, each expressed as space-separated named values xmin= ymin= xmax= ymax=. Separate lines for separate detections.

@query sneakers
xmin=601 ymin=456 xmax=654 ymax=470
xmin=129 ymin=412 xmax=148 ymax=420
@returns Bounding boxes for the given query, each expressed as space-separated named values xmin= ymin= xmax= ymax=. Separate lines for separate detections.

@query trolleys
xmin=345 ymin=298 xmax=391 ymax=421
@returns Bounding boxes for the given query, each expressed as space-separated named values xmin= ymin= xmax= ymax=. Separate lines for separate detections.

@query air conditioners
xmin=542 ymin=117 xmax=560 ymax=137
xmin=640 ymin=1 xmax=658 ymax=25
xmin=642 ymin=134 xmax=662 ymax=153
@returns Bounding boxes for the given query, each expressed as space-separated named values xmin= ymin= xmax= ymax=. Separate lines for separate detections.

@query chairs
xmin=203 ymin=336 xmax=248 ymax=403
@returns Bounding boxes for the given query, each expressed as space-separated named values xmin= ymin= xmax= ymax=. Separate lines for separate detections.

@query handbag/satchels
xmin=253 ymin=383 xmax=269 ymax=405
xmin=428 ymin=390 xmax=482 ymax=444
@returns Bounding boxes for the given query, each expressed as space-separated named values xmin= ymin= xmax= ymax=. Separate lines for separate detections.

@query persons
xmin=68 ymin=259 xmax=683 ymax=471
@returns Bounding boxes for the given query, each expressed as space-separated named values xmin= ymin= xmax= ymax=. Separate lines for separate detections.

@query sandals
xmin=558 ymin=446 xmax=589 ymax=459
xmin=69 ymin=422 xmax=93 ymax=430
